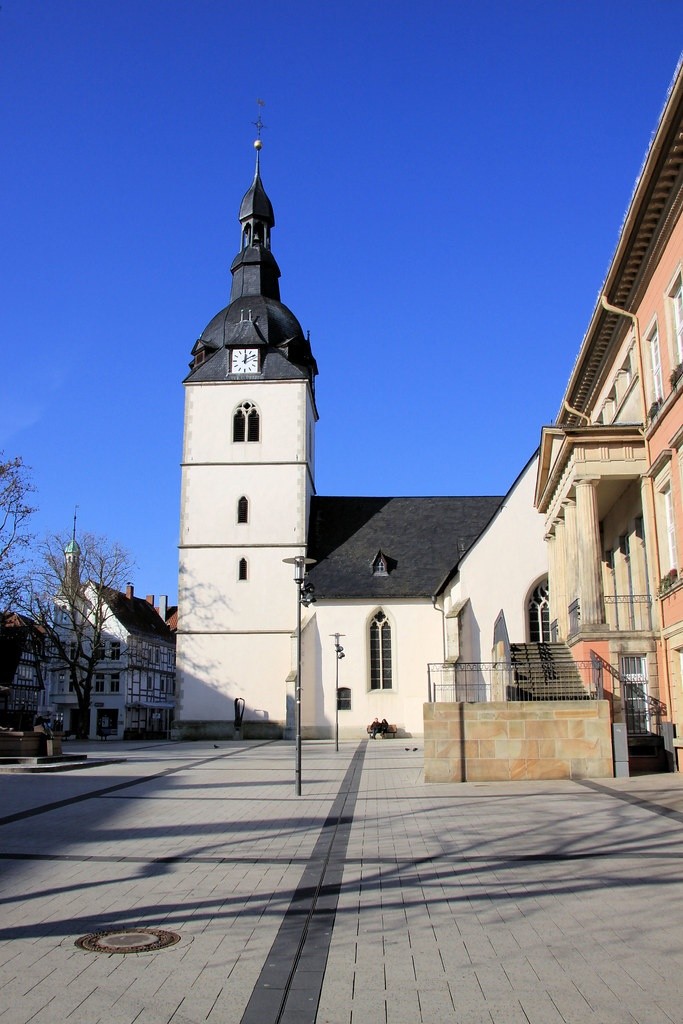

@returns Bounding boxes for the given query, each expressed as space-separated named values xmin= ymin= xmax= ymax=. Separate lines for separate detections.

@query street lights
xmin=284 ymin=555 xmax=318 ymax=796
xmin=329 ymin=632 xmax=346 ymax=752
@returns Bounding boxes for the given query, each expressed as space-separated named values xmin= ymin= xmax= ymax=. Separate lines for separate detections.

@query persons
xmin=370 ymin=718 xmax=381 ymax=739
xmin=379 ymin=719 xmax=388 ymax=739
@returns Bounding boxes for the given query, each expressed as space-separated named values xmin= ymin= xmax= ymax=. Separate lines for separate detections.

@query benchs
xmin=367 ymin=724 xmax=397 ymax=738
